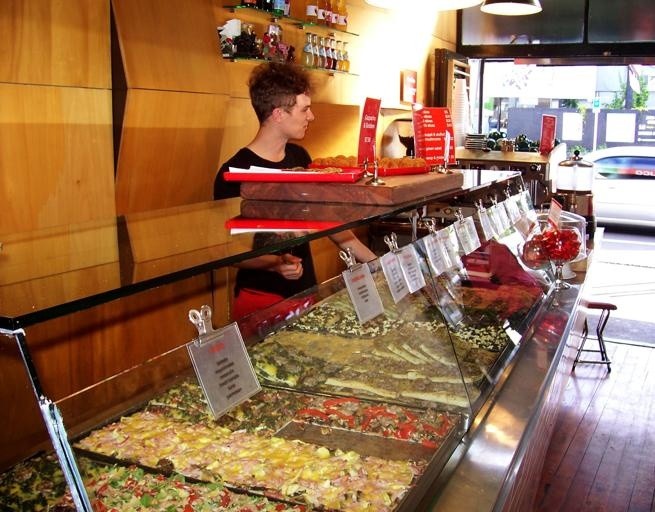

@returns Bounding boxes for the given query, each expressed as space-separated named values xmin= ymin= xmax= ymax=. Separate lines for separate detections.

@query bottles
xmin=304 ymin=0 xmax=348 ymax=33
xmin=241 ymin=0 xmax=291 ymax=19
xmin=301 ymin=32 xmax=351 ymax=71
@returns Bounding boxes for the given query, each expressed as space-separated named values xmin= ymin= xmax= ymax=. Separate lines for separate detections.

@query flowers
xmin=542 ymin=229 xmax=581 ymax=261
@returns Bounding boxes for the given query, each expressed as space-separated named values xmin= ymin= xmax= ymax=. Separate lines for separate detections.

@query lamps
xmin=551 ymin=258 xmax=571 ymax=290
xmin=480 ymin=0 xmax=543 ymax=17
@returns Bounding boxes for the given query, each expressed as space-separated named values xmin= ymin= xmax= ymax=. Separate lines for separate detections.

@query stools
xmin=572 ymin=300 xmax=618 ymax=371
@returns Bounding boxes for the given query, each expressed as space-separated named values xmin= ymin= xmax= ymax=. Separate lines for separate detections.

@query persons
xmin=213 ymin=60 xmax=377 ymax=340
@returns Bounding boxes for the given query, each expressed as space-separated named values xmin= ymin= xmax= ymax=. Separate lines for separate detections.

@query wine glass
xmin=533 ymin=290 xmax=571 ymax=349
xmin=517 ymin=230 xmax=556 ymax=283
xmin=540 ymin=226 xmax=583 ymax=290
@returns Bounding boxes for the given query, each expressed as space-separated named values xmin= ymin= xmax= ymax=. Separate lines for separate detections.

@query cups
xmin=452 ymin=77 xmax=469 ymax=148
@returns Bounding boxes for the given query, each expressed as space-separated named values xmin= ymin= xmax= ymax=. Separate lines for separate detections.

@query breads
xmin=312 ymin=155 xmax=427 ymax=169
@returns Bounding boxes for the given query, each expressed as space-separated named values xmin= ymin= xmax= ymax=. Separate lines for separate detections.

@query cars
xmin=547 ymin=145 xmax=655 ymax=231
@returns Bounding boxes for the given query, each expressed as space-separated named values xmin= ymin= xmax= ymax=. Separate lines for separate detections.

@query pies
xmin=326 ymin=322 xmax=496 ymax=406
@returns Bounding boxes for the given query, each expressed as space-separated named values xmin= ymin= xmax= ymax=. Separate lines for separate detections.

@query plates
xmin=463 ymin=133 xmax=487 ymax=149
